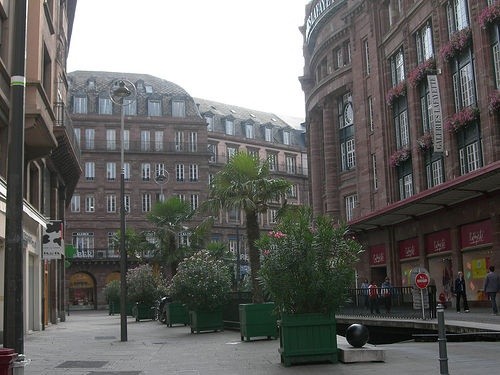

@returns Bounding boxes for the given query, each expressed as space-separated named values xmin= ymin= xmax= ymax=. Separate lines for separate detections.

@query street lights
xmin=107 ymin=78 xmax=137 ymax=343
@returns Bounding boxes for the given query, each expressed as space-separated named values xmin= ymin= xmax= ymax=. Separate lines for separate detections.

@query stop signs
xmin=415 ymin=273 xmax=430 ymax=289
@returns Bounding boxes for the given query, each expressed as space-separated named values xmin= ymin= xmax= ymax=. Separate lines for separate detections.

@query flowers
xmin=102 ymin=279 xmax=122 ymax=300
xmin=407 ymin=56 xmax=435 ymax=89
xmin=487 ymin=86 xmax=500 ymax=116
xmin=438 ymin=28 xmax=471 ymax=64
xmin=254 ymin=200 xmax=366 ymax=320
xmin=168 ymin=248 xmax=232 ymax=313
xmin=389 ymin=146 xmax=410 ymax=170
xmin=476 ymin=0 xmax=500 ymax=30
xmin=386 ymin=79 xmax=406 ymax=107
xmin=445 ymin=105 xmax=480 ymax=135
xmin=127 ymin=263 xmax=167 ymax=302
xmin=417 ymin=131 xmax=433 ymax=153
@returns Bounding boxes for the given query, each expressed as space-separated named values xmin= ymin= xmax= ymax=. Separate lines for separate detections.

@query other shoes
xmin=464 ymin=310 xmax=470 ymax=312
xmin=458 ymin=312 xmax=460 ymax=313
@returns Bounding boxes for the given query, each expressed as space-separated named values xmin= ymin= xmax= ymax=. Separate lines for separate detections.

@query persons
xmin=361 ymin=279 xmax=368 ymax=294
xmin=484 ymin=266 xmax=498 ymax=315
xmin=455 ymin=271 xmax=471 ymax=313
xmin=381 ymin=277 xmax=391 ymax=313
xmin=368 ymin=281 xmax=381 ymax=314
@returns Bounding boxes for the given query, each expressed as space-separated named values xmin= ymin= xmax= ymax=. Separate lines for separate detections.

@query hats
xmin=458 ymin=271 xmax=463 ymax=275
xmin=384 ymin=277 xmax=390 ymax=280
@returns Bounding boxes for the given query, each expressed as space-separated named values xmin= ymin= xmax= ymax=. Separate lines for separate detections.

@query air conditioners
xmin=97 ymin=250 xmax=106 ymax=257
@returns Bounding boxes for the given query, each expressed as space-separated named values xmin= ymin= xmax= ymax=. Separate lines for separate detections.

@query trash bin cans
xmin=0 ymin=349 xmax=19 ymax=375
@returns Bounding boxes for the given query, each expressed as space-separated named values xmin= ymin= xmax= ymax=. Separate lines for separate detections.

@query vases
xmin=188 ymin=303 xmax=225 ymax=334
xmin=133 ymin=302 xmax=157 ymax=322
xmin=108 ymin=298 xmax=122 ymax=317
xmin=276 ymin=309 xmax=338 ymax=367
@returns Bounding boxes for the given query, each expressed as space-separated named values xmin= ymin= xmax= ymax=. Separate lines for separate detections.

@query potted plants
xmin=142 ymin=193 xmax=213 ymax=327
xmin=198 ymin=148 xmax=294 ymax=342
